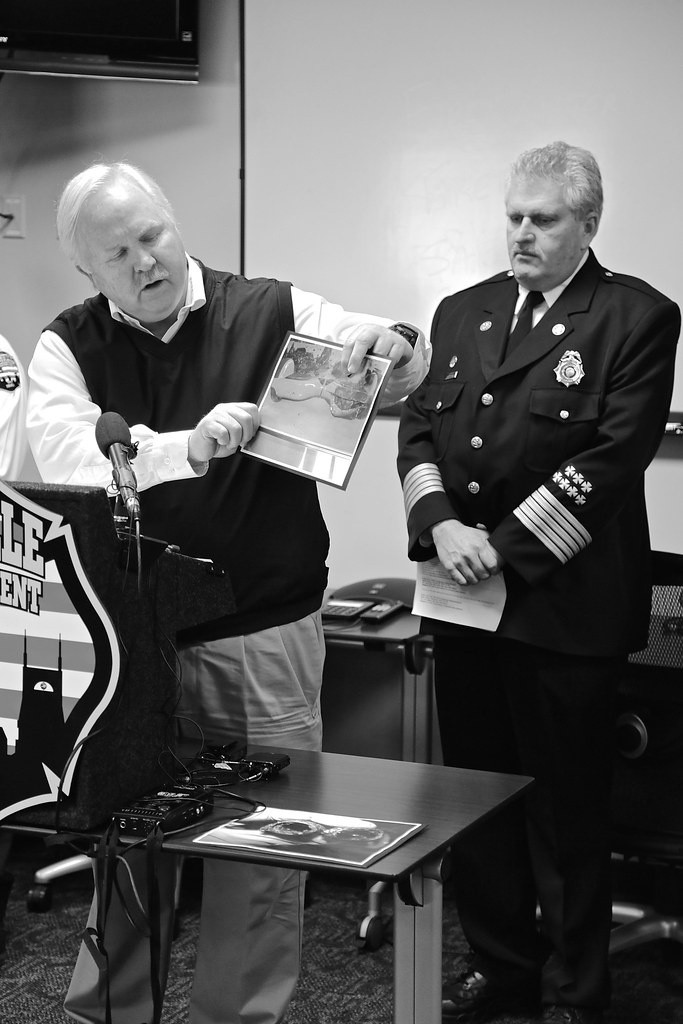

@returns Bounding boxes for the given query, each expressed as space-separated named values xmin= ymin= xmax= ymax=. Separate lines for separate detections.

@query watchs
xmin=390 ymin=322 xmax=418 ymax=346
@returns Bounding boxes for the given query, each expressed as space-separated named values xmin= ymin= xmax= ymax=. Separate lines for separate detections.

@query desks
xmin=322 ymin=614 xmax=683 ymax=952
xmin=0 ymin=735 xmax=537 ymax=1024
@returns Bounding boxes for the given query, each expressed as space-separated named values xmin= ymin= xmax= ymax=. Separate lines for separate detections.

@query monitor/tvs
xmin=0 ymin=0 xmax=200 ymax=84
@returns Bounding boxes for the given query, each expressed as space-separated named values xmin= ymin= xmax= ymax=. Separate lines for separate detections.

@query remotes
xmin=360 ymin=602 xmax=403 ymax=624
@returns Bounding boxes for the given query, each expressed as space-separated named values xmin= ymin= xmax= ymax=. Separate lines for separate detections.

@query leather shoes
xmin=441 ymin=969 xmax=543 ymax=1018
xmin=542 ymin=1005 xmax=603 ymax=1024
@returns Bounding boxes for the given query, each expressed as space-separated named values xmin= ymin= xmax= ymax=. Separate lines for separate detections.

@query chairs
xmin=537 ymin=550 xmax=683 ymax=953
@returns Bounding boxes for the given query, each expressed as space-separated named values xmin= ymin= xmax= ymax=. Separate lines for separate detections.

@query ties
xmin=504 ymin=291 xmax=545 ymax=362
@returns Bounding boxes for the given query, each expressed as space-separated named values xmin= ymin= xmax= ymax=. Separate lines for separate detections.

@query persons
xmin=395 ymin=142 xmax=682 ymax=1024
xmin=25 ymin=158 xmax=424 ymax=1024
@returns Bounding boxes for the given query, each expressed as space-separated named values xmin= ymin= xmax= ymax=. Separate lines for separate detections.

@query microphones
xmin=95 ymin=412 xmax=142 ymax=523
